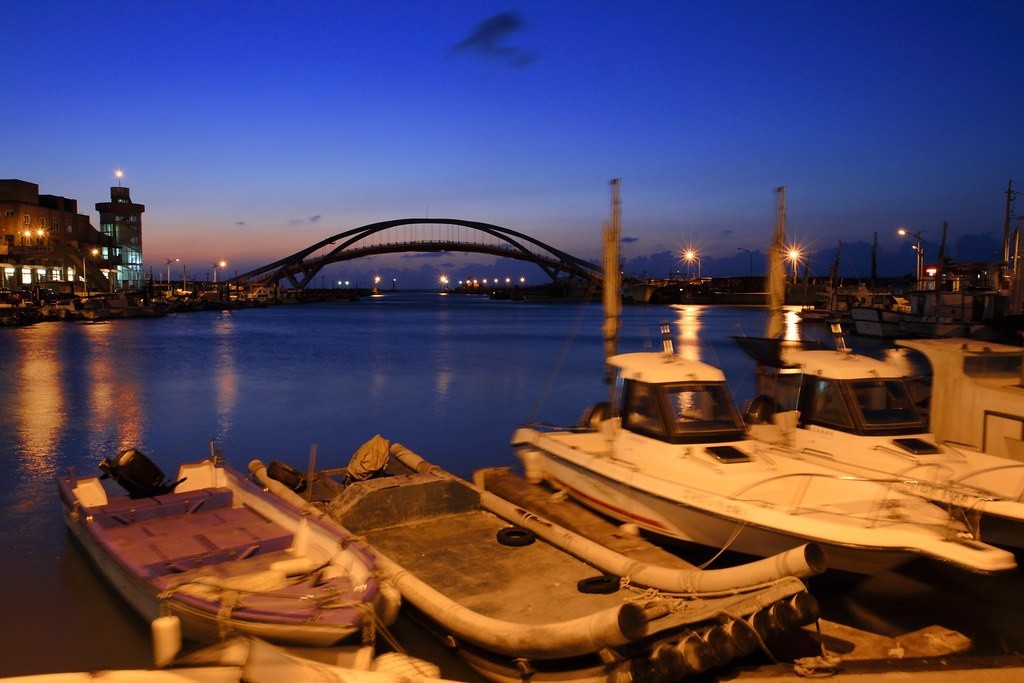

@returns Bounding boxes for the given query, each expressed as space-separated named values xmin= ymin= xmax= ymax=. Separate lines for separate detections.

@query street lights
xmin=83 ymin=249 xmax=98 ymax=296
xmin=687 ymin=253 xmax=700 ymax=277
xmin=897 ymin=230 xmax=924 ymax=289
xmin=790 ymin=252 xmax=799 ymax=285
xmin=165 ymin=258 xmax=179 ymax=297
xmin=737 ymin=247 xmax=752 ymax=276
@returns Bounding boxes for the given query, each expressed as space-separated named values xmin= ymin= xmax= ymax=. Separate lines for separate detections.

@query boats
xmin=628 ymin=271 xmax=689 ymax=305
xmin=58 ymin=449 xmax=382 ymax=650
xmin=510 ymin=323 xmax=1024 ymax=637
xmin=794 ymin=284 xmax=910 ymax=320
xmin=489 ymin=285 xmax=522 ymax=301
xmin=247 ymin=434 xmax=830 ymax=683
xmin=851 ymin=289 xmax=1023 ymax=324
